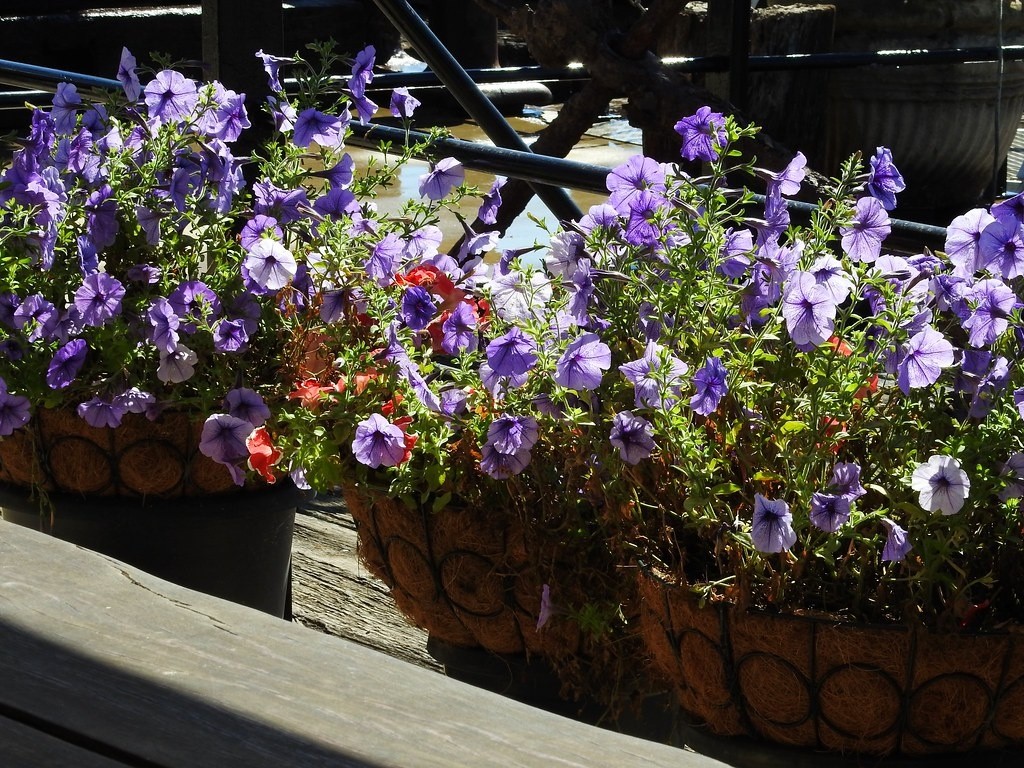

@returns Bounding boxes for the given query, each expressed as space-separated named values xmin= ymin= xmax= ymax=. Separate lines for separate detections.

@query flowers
xmin=1 ymin=47 xmax=1024 ymax=624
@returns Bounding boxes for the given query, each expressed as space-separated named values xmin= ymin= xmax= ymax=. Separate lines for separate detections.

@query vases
xmin=634 ymin=561 xmax=1024 ymax=751
xmin=338 ymin=477 xmax=636 ymax=657
xmin=2 ymin=392 xmax=289 ymax=495
